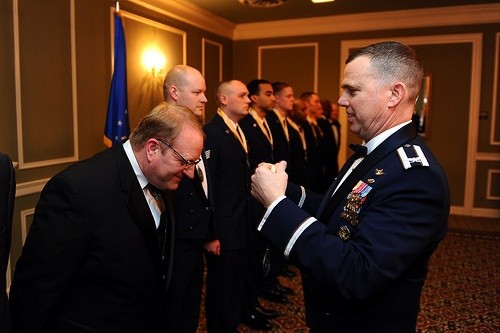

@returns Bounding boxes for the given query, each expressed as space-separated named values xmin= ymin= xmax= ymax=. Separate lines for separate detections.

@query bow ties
xmin=348 ymin=143 xmax=367 ymax=158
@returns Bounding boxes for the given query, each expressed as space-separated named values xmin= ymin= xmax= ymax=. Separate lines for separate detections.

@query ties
xmin=144 ymin=181 xmax=165 ymax=214
xmin=196 ymin=164 xmax=204 ymax=183
xmin=237 ymin=126 xmax=243 ymax=143
xmin=262 ymin=121 xmax=270 ymax=139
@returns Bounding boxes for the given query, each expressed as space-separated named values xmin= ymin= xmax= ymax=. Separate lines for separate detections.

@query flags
xmin=102 ymin=14 xmax=131 ymax=147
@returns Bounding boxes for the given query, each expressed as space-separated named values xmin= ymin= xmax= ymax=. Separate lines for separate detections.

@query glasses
xmin=156 ymin=138 xmax=201 ymax=167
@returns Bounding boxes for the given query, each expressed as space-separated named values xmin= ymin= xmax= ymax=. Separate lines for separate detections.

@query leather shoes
xmin=239 ymin=264 xmax=298 ymax=333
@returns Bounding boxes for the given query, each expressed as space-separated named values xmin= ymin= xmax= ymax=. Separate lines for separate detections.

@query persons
xmin=162 ymin=64 xmax=215 ymax=333
xmin=249 ymin=41 xmax=451 ymax=333
xmin=7 ymin=101 xmax=205 ymax=333
xmin=201 ymin=74 xmax=343 ymax=333
